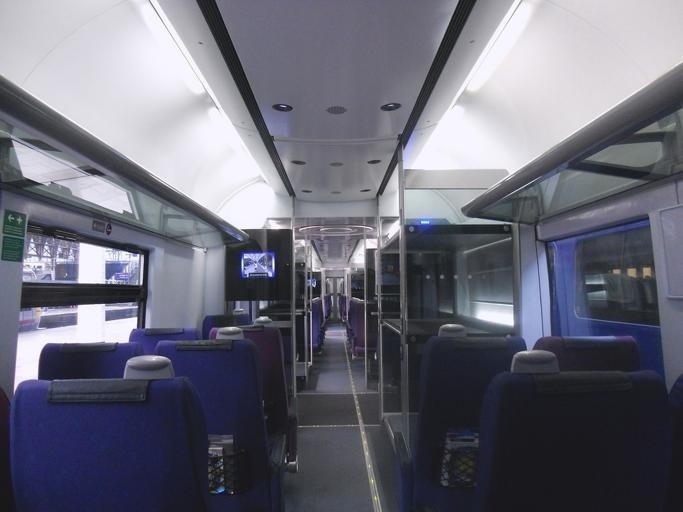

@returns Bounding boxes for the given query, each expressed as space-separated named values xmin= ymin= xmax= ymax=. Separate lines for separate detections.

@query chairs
xmin=0 ymin=315 xmax=297 ymax=510
xmin=399 ymin=324 xmax=682 ymax=512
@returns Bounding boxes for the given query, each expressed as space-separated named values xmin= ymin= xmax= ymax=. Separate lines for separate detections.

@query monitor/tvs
xmin=239 ymin=251 xmax=276 ymax=279
xmin=308 ymin=276 xmax=317 ymax=290
